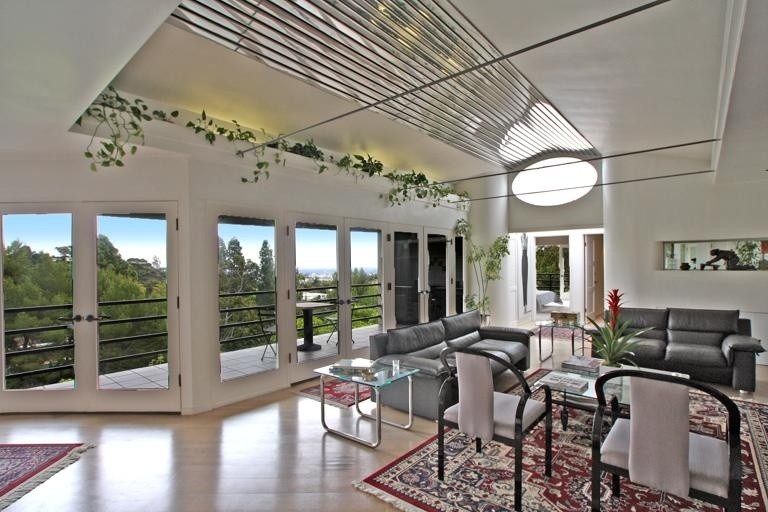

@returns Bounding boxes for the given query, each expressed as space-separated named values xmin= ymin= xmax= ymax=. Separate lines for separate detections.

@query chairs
xmin=436 ymin=348 xmax=552 ymax=510
xmin=591 ymin=370 xmax=741 ymax=512
xmin=258 ymin=306 xmax=278 ymax=360
xmin=328 ymin=302 xmax=354 ymax=346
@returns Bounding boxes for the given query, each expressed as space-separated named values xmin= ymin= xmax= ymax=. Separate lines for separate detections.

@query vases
xmin=598 ymin=362 xmax=624 ymax=384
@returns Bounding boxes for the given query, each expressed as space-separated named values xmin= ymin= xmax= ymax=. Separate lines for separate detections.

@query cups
xmin=393 ymin=359 xmax=399 ymax=370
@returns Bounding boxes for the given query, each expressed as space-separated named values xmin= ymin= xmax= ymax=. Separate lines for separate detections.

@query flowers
xmin=573 ymin=289 xmax=655 ymax=385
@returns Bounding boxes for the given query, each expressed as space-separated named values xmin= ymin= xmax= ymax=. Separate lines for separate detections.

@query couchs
xmin=370 ymin=305 xmax=535 ymax=423
xmin=586 ymin=306 xmax=765 ymax=391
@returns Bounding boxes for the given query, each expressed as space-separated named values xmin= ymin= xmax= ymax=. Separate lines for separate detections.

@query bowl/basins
xmin=362 ymin=368 xmax=375 ymax=378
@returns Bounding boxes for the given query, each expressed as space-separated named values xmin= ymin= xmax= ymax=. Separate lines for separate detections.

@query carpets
xmin=356 ymin=356 xmax=766 ymax=511
xmin=0 ymin=443 xmax=96 ymax=512
xmin=288 ymin=372 xmax=372 ymax=408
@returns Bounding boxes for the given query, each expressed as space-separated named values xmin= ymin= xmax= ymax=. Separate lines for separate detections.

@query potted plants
xmin=454 ymin=218 xmax=510 ymax=328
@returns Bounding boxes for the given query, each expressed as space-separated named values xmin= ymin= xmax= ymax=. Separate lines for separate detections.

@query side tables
xmin=312 ymin=356 xmax=418 ymax=448
xmin=536 ymin=320 xmax=590 ymax=360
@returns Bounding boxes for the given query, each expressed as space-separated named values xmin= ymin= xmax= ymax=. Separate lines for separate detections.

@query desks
xmin=297 ymin=300 xmax=332 ymax=352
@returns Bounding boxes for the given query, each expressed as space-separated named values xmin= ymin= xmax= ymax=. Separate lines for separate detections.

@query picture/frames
xmin=656 ymin=237 xmax=768 ymax=270
